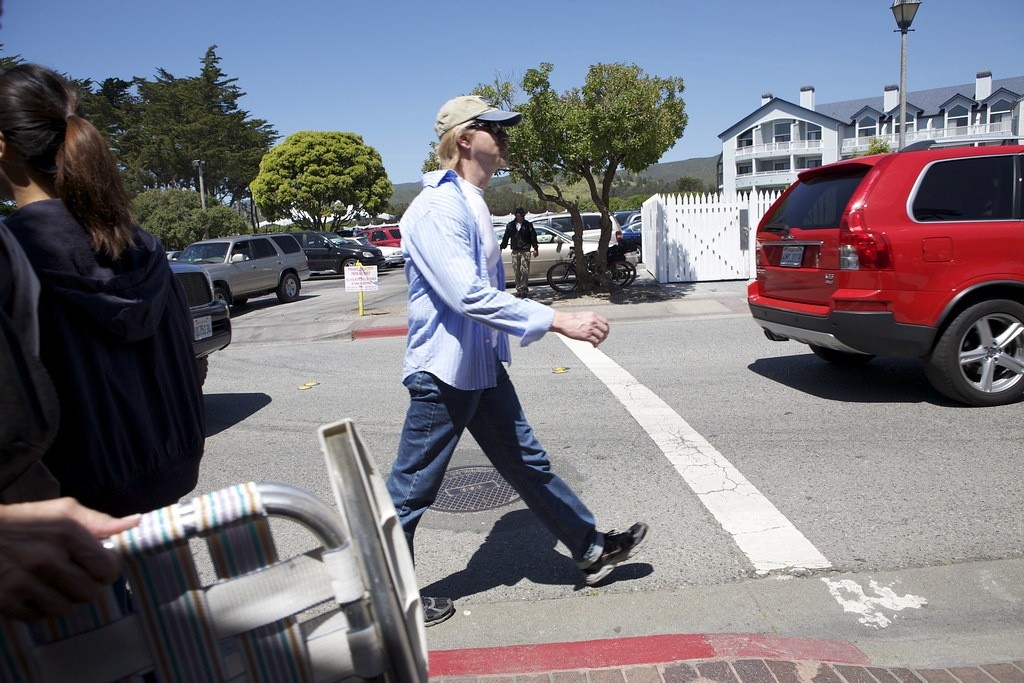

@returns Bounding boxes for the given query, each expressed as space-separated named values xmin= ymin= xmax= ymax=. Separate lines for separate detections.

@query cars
xmin=309 ymin=237 xmax=374 ymax=274
xmin=290 ymin=232 xmax=385 ymax=274
xmin=376 ymin=246 xmax=405 ymax=268
xmin=167 ymin=260 xmax=232 ymax=387
xmin=166 ymin=251 xmax=203 ymax=262
xmin=493 ymin=224 xmax=638 ymax=283
xmin=621 ymin=220 xmax=642 ymax=263
xmin=612 ymin=211 xmax=641 ymax=232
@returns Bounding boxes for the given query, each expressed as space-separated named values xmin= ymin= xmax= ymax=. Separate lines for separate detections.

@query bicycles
xmin=547 ymin=246 xmax=636 ymax=293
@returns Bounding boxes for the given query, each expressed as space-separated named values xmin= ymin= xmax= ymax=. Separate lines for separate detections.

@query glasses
xmin=465 ymin=121 xmax=503 ymax=135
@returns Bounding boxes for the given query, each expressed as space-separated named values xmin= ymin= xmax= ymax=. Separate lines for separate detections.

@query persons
xmin=0 ymin=64 xmax=206 ymax=519
xmin=0 ymin=220 xmax=142 ymax=625
xmin=387 ymin=95 xmax=651 ymax=627
xmin=500 ymin=208 xmax=539 ymax=299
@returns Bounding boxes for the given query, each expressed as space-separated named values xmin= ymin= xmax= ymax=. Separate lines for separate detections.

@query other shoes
xmin=521 ymin=294 xmax=528 ymax=299
xmin=511 ymin=293 xmax=522 ymax=297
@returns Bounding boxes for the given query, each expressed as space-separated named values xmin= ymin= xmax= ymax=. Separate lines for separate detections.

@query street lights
xmin=889 ymin=0 xmax=922 ymax=152
xmin=193 ymin=159 xmax=209 ymax=239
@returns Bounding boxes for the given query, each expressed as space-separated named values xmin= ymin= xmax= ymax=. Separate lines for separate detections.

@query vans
xmin=528 ymin=212 xmax=623 ymax=246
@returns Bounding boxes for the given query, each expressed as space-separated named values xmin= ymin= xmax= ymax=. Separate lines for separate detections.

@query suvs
xmin=351 ymin=227 xmax=402 ymax=247
xmin=747 ymin=136 xmax=1024 ymax=407
xmin=174 ymin=234 xmax=311 ymax=309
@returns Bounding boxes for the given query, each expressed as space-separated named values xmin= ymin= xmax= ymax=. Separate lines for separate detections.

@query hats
xmin=515 ymin=207 xmax=525 ymax=217
xmin=434 ymin=96 xmax=522 ymax=140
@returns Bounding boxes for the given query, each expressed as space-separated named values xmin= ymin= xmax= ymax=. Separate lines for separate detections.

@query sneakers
xmin=421 ymin=596 xmax=455 ymax=628
xmin=572 ymin=522 xmax=649 ymax=586
